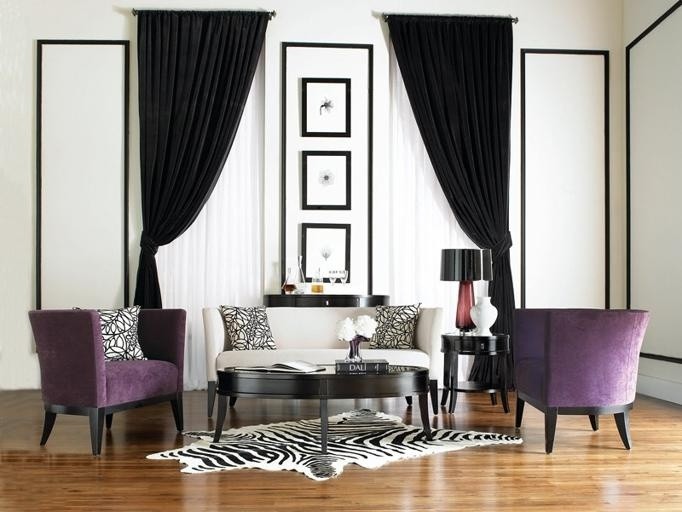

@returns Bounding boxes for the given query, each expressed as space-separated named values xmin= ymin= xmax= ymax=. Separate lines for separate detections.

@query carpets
xmin=145 ymin=407 xmax=524 ymax=481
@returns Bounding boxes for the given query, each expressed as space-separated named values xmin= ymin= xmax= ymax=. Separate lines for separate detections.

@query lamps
xmin=440 ymin=248 xmax=493 ymax=333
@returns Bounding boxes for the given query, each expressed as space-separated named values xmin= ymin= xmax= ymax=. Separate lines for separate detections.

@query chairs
xmin=28 ymin=308 xmax=187 ymax=455
xmin=510 ymin=308 xmax=650 ymax=455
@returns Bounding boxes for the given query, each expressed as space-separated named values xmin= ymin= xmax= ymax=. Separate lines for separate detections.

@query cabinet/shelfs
xmin=264 ymin=294 xmax=390 ymax=307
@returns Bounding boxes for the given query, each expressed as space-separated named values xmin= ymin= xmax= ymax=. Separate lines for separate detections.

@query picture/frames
xmin=301 ymin=77 xmax=352 ymax=284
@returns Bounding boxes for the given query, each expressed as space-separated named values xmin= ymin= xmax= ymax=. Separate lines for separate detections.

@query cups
xmin=311 ymin=272 xmax=324 ymax=293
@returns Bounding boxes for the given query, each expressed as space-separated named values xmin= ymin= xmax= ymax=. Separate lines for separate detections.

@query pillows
xmin=219 ymin=304 xmax=279 ymax=351
xmin=71 ymin=305 xmax=149 ymax=364
xmin=369 ymin=301 xmax=422 ymax=350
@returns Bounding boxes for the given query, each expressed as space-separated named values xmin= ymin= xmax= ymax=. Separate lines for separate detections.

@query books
xmin=334 ymin=359 xmax=390 ymax=373
xmin=234 ymin=359 xmax=326 ymax=374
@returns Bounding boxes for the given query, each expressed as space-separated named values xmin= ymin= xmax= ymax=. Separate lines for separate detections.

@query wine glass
xmin=329 ymin=271 xmax=349 ymax=294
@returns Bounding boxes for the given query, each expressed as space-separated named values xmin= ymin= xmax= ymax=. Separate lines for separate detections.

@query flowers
xmin=335 ymin=314 xmax=378 ymax=342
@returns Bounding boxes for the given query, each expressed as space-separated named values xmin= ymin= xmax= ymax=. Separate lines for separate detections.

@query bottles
xmin=281 ymin=255 xmax=306 ymax=294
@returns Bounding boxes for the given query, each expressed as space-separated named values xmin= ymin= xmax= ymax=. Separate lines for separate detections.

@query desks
xmin=440 ymin=334 xmax=511 ymax=414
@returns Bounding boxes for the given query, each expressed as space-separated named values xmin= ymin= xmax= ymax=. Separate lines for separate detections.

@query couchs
xmin=201 ymin=305 xmax=445 ymax=418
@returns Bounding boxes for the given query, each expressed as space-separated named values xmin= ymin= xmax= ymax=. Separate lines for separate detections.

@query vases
xmin=345 ymin=335 xmax=370 ymax=362
xmin=469 ymin=296 xmax=499 ymax=336
